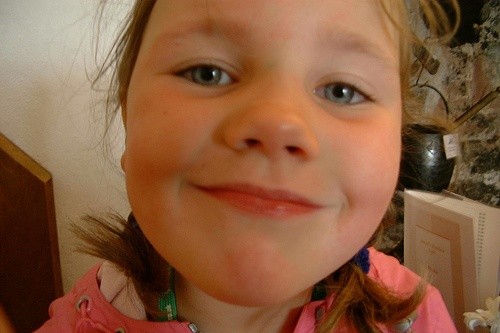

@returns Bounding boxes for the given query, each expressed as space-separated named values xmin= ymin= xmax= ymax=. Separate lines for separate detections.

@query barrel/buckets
xmin=395 ymin=82 xmax=457 ymax=194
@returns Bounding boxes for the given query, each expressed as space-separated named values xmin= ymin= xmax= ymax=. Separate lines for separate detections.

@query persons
xmin=30 ymin=0 xmax=461 ymax=333
